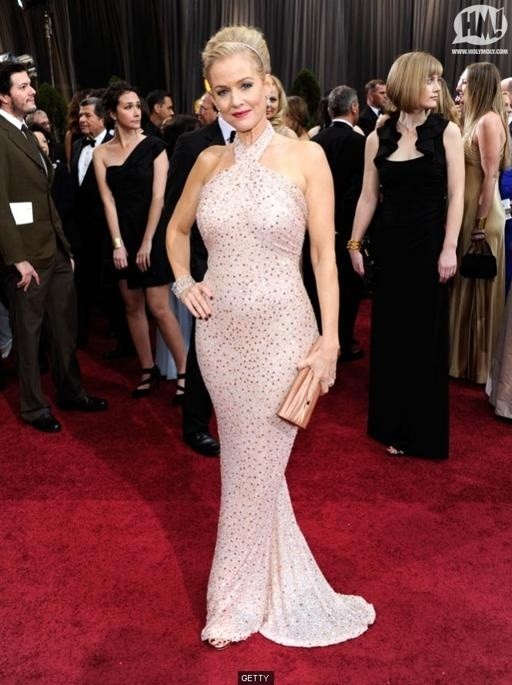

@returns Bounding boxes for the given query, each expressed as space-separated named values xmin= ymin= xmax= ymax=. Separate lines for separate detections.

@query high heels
xmin=135 ymin=365 xmax=160 ymax=397
xmin=176 ymin=373 xmax=185 ymax=399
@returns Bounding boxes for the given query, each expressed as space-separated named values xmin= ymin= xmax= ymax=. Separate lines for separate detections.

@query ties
xmin=21 ymin=125 xmax=37 ymax=148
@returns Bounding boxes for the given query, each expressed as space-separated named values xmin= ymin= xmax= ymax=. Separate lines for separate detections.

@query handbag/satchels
xmin=459 ymin=253 xmax=497 ymax=281
xmin=276 ymin=335 xmax=341 ymax=430
xmin=361 ymin=237 xmax=376 ymax=282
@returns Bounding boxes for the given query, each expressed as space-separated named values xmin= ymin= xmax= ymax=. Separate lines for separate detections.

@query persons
xmin=0 ymin=49 xmax=512 ymax=460
xmin=165 ymin=23 xmax=376 ymax=652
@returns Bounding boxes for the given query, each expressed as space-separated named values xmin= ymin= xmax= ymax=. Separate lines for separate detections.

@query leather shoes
xmin=57 ymin=395 xmax=107 ymax=412
xmin=343 ymin=350 xmax=362 ymax=361
xmin=182 ymin=433 xmax=219 ymax=455
xmin=22 ymin=411 xmax=61 ymax=433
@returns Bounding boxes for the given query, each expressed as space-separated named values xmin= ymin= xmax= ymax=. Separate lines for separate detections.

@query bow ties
xmin=81 ymin=138 xmax=96 ymax=148
